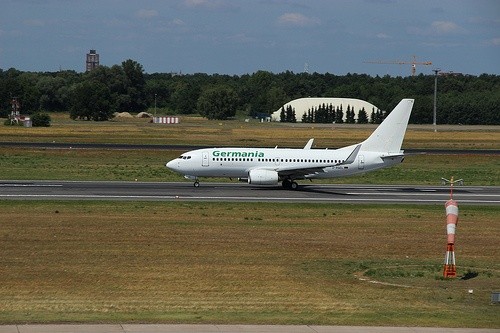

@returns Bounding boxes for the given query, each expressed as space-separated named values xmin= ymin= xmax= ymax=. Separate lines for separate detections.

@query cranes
xmin=365 ymin=54 xmax=433 ymax=76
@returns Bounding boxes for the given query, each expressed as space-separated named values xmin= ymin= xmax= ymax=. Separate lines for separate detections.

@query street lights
xmin=155 ymin=93 xmax=158 ymax=116
xmin=431 ymin=69 xmax=441 ymax=131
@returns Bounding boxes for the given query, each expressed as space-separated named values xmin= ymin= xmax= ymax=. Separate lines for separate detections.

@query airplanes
xmin=166 ymin=98 xmax=414 ymax=188
